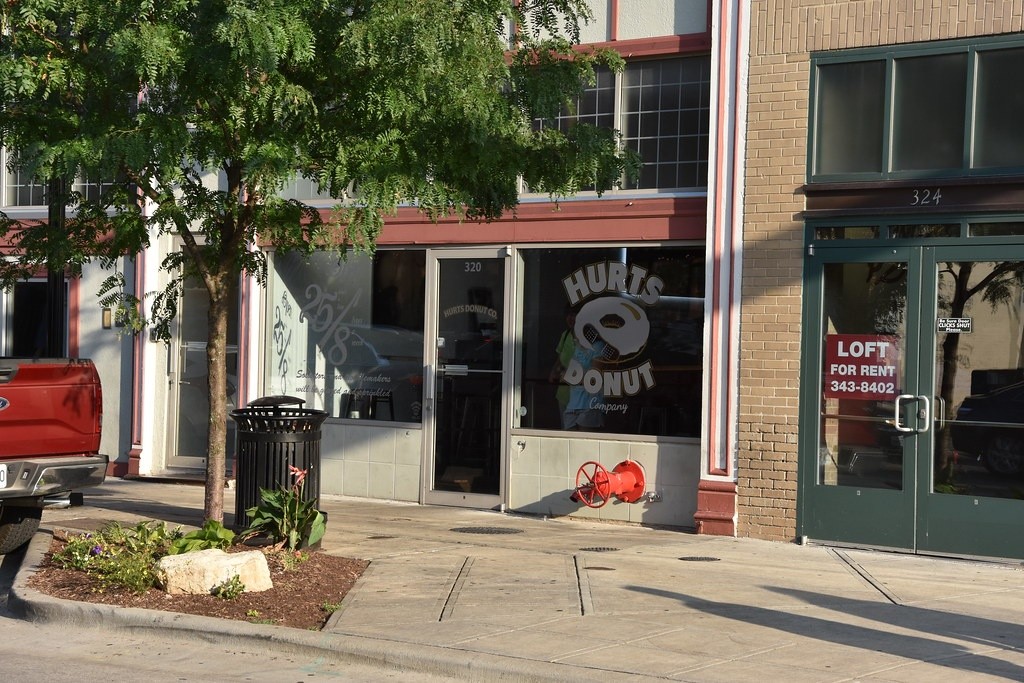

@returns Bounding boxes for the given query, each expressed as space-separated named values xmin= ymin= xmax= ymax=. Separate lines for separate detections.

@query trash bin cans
xmin=229 ymin=395 xmax=330 ymax=550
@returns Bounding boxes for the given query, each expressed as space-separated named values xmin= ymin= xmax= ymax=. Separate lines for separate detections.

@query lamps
xmin=115 ymin=309 xmax=129 ymax=327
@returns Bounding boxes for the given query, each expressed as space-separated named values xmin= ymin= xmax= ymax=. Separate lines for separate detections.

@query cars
xmin=948 ymin=380 xmax=1024 ymax=484
xmin=817 ymin=370 xmax=907 ymax=451
xmin=312 ymin=317 xmax=423 ymax=424
xmin=179 ymin=341 xmax=239 ymax=456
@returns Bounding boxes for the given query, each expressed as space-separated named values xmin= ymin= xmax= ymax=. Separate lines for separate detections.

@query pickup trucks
xmin=0 ymin=352 xmax=110 ymax=559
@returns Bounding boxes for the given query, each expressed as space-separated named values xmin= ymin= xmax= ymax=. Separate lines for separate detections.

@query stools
xmin=344 ymin=389 xmax=396 ymax=421
